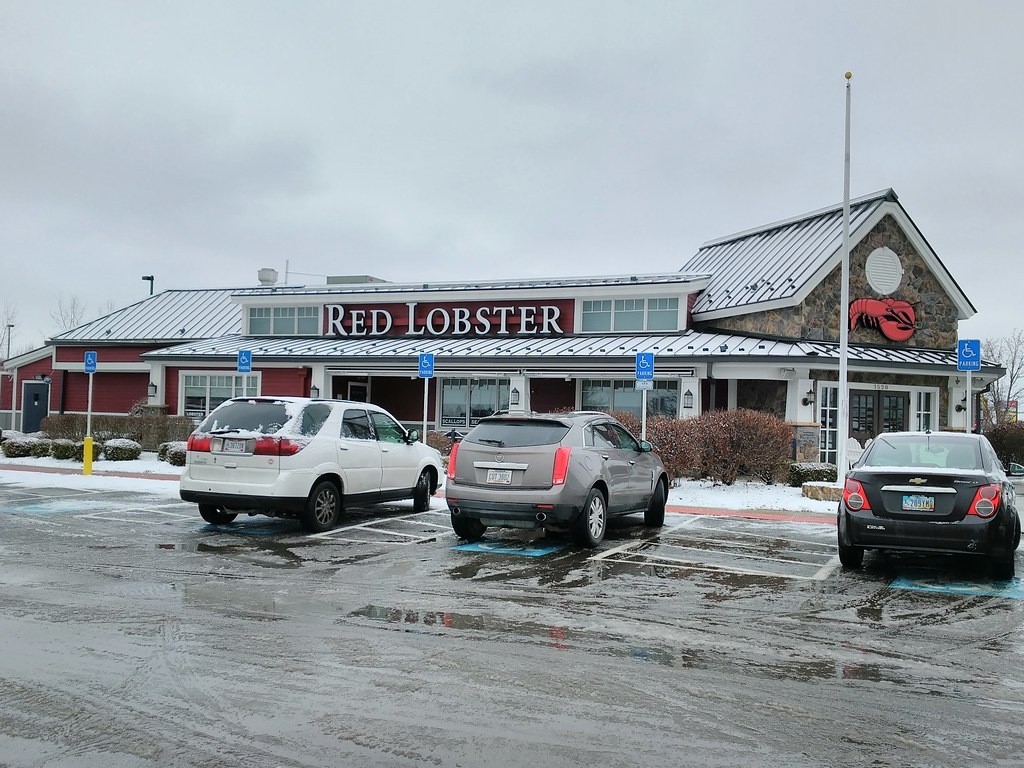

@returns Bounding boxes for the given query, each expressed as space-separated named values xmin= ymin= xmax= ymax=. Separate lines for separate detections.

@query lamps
xmin=41 ymin=373 xmax=52 ymax=385
xmin=309 ymin=385 xmax=319 ymax=398
xmin=683 ymin=389 xmax=693 ymax=408
xmin=511 ymin=387 xmax=519 ymax=405
xmin=801 ymin=389 xmax=814 ymax=406
xmin=955 ymin=396 xmax=966 ymax=412
xmin=148 ymin=382 xmax=157 ymax=397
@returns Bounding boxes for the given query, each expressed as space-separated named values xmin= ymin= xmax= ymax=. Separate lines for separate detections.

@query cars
xmin=836 ymin=433 xmax=1020 ymax=583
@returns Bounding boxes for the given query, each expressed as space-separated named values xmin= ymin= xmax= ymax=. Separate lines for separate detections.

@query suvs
xmin=179 ymin=396 xmax=445 ymax=532
xmin=445 ymin=409 xmax=669 ymax=548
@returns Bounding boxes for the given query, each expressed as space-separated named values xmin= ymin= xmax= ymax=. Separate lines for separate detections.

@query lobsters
xmin=848 ymin=298 xmax=918 ymax=343
